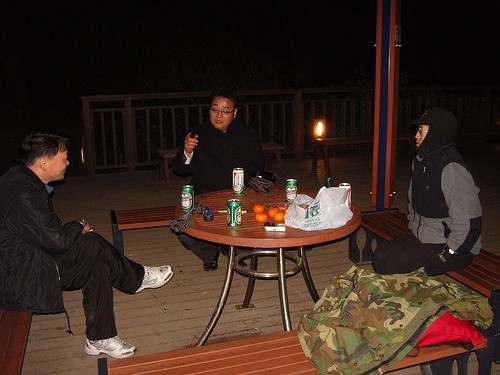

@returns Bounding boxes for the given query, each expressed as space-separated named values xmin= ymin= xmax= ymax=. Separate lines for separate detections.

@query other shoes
xmin=204 ymin=246 xmax=220 ymax=272
xmin=220 ymin=245 xmax=247 ymax=266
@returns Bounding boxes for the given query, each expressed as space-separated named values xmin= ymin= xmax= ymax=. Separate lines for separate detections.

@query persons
xmin=177 ymin=88 xmax=269 ymax=270
xmin=0 ymin=133 xmax=174 ymax=357
xmin=371 ymin=108 xmax=482 ymax=275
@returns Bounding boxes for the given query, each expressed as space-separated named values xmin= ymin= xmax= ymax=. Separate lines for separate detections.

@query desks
xmin=176 ymin=188 xmax=362 ymax=346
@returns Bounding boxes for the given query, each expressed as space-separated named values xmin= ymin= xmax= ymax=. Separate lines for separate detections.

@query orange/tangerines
xmin=254 ymin=204 xmax=289 ymax=222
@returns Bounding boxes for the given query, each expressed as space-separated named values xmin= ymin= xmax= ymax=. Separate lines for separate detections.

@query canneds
xmin=226 ymin=199 xmax=242 ymax=227
xmin=285 ymin=179 xmax=297 ymax=203
xmin=180 ymin=185 xmax=194 ymax=212
xmin=338 ymin=182 xmax=352 ymax=208
xmin=232 ymin=168 xmax=245 ymax=195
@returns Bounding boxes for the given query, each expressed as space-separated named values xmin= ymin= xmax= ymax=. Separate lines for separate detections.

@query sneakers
xmin=135 ymin=264 xmax=174 ymax=293
xmin=85 ymin=335 xmax=137 ymax=358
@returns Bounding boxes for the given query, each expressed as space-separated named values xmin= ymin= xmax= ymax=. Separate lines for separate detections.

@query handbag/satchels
xmin=284 ymin=185 xmax=353 ymax=231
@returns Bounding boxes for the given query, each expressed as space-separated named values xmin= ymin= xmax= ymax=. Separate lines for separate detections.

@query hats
xmin=409 ymin=107 xmax=457 ymax=125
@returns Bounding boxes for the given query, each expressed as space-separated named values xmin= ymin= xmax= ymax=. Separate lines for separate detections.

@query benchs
xmin=0 ymin=306 xmax=32 ymax=375
xmin=157 ymin=141 xmax=284 ymax=187
xmin=309 ymin=137 xmax=415 ymax=179
xmin=358 ymin=207 xmax=500 ymax=324
xmin=110 ymin=207 xmax=176 ymax=256
xmin=97 ymin=320 xmax=499 ymax=375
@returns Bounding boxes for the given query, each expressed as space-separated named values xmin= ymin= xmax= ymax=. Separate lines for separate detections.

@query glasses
xmin=209 ymin=105 xmax=234 ymax=114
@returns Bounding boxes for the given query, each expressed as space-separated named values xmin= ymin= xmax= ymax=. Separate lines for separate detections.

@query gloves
xmin=436 ymin=248 xmax=455 ymax=268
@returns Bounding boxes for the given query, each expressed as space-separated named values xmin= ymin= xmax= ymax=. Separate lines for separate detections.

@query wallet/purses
xmin=248 ymin=168 xmax=276 ymax=193
